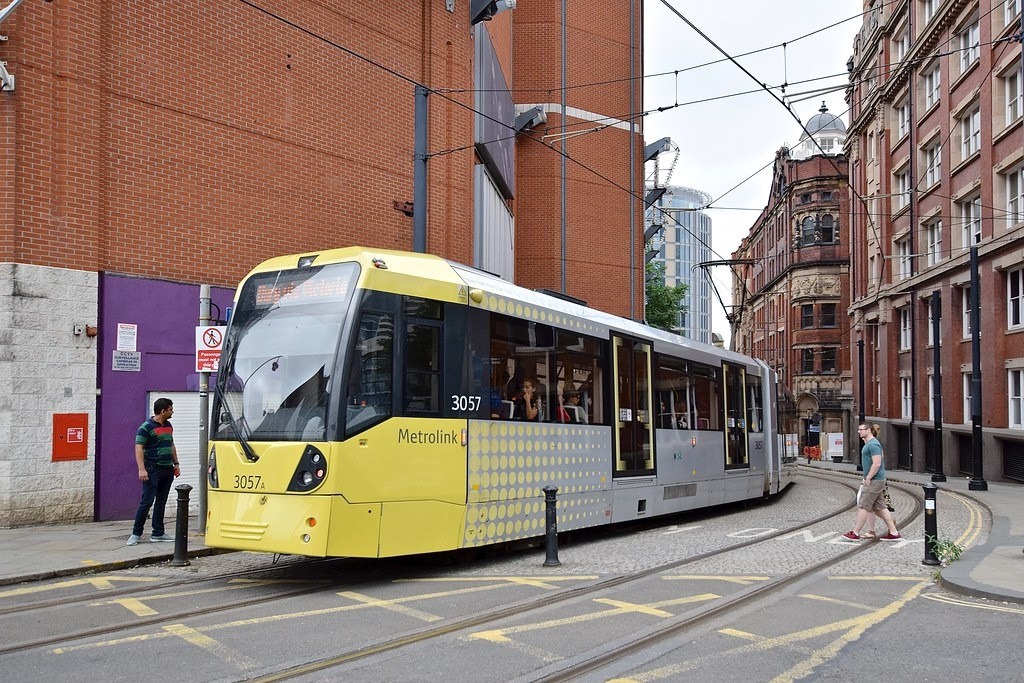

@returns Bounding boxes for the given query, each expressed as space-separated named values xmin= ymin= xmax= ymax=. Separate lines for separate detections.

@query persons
xmin=562 ymin=390 xmax=589 ymax=423
xmin=125 ymin=399 xmax=181 ymax=546
xmin=656 ymin=401 xmax=704 ymax=429
xmin=841 ymin=421 xmax=902 ymax=543
xmin=803 ymin=445 xmax=822 ymax=461
xmin=577 ymin=372 xmax=593 ymax=414
xmin=506 ymin=364 xmax=526 ymax=419
xmin=514 ymin=378 xmax=540 ymax=422
xmin=528 ymin=375 xmax=546 ymax=408
xmin=562 ymin=379 xmax=577 ymax=392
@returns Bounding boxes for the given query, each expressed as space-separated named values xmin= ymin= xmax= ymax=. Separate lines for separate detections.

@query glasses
xmin=858 ymin=429 xmax=867 ymax=431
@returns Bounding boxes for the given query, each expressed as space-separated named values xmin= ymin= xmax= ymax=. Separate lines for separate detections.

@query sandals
xmin=861 ymin=531 xmax=876 ymax=539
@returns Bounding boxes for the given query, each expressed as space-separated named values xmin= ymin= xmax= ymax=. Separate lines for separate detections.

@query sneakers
xmin=150 ymin=534 xmax=175 ymax=542
xmin=842 ymin=531 xmax=859 ymax=541
xmin=126 ymin=534 xmax=140 ymax=546
xmin=879 ymin=532 xmax=901 ymax=541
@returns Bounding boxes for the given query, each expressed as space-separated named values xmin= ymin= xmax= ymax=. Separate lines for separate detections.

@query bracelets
xmin=174 ymin=462 xmax=179 ymax=465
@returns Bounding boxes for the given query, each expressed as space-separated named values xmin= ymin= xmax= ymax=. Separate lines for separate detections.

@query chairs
xmin=497 ymin=400 xmax=709 ymax=430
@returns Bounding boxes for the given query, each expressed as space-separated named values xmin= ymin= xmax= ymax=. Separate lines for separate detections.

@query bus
xmin=200 ymin=246 xmax=799 ymax=560
